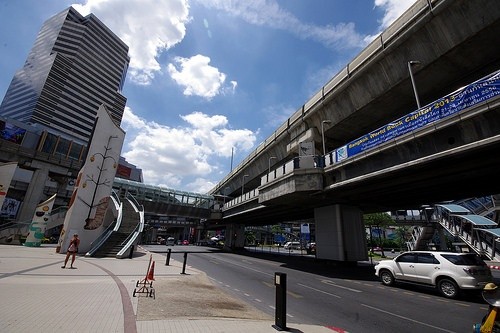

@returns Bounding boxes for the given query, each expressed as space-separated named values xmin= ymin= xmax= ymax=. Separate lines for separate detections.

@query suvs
xmin=374 ymin=250 xmax=493 ymax=299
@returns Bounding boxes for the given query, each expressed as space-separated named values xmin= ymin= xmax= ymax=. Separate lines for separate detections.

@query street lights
xmin=268 ymin=156 xmax=278 ymax=180
xmin=223 ymin=186 xmax=230 ymax=204
xmin=242 ymin=174 xmax=249 ymax=195
xmin=321 ymin=119 xmax=332 ymax=155
xmin=406 ymin=58 xmax=422 ymax=111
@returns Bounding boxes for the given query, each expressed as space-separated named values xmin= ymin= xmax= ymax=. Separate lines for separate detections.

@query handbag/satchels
xmin=69 ymin=243 xmax=77 ymax=252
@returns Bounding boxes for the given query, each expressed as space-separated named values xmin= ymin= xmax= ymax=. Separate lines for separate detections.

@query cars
xmin=156 ymin=231 xmax=404 ymax=257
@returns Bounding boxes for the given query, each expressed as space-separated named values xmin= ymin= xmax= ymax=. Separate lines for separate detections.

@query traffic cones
xmin=147 ymin=261 xmax=155 ymax=280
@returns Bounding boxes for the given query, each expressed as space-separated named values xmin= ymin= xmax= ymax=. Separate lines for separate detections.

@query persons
xmin=61 ymin=233 xmax=80 ymax=268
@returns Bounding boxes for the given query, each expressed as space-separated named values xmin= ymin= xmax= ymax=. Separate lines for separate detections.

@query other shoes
xmin=61 ymin=266 xmax=65 ymax=269
